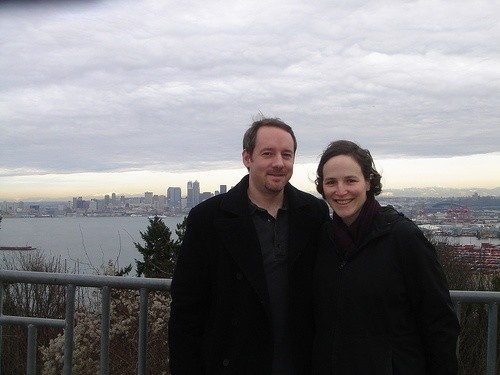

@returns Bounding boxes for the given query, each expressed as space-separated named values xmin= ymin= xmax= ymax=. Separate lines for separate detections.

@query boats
xmin=0 ymin=246 xmax=36 ymax=250
xmin=147 ymin=214 xmax=168 ymax=218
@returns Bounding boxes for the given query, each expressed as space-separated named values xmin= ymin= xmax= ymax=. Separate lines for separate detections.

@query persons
xmin=166 ymin=117 xmax=332 ymax=374
xmin=315 ymin=139 xmax=466 ymax=375
